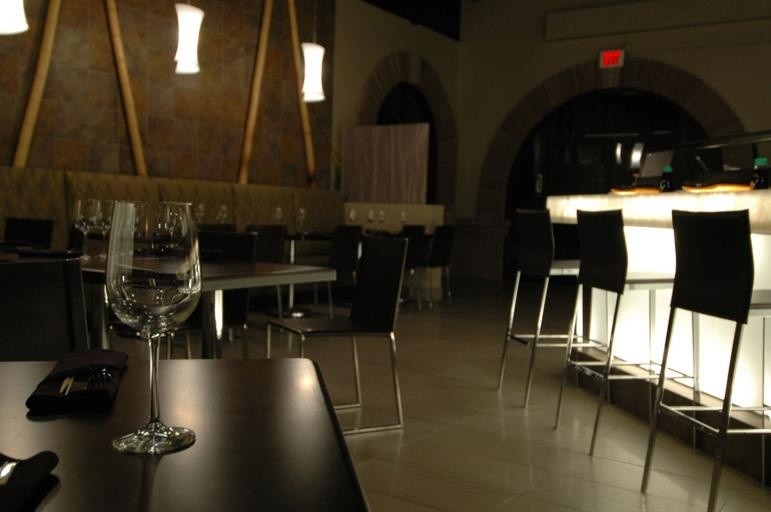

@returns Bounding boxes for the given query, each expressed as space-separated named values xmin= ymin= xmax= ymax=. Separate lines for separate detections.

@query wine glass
xmin=103 ymin=198 xmax=202 ymax=454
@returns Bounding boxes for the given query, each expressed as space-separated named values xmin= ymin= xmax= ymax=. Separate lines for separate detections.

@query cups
xmin=73 ymin=198 xmax=115 ymax=262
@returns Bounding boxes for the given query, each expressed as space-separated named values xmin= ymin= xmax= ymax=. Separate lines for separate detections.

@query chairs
xmin=327 ymin=226 xmax=363 ymax=294
xmin=423 ymin=234 xmax=438 ymax=308
xmin=264 ymin=230 xmax=409 ymax=433
xmin=553 ymin=210 xmax=704 ymax=456
xmin=1 ymin=220 xmax=456 ymax=279
xmin=433 ymin=223 xmax=456 ymax=303
xmin=398 ymin=224 xmax=425 ymax=313
xmin=166 ymin=230 xmax=257 ymax=357
xmin=497 ymin=209 xmax=614 ymax=409
xmin=638 ymin=209 xmax=771 ymax=512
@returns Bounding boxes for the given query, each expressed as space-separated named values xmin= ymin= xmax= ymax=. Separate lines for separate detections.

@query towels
xmin=0 ymin=450 xmax=60 ymax=512
xmin=26 ymin=351 xmax=129 ymax=412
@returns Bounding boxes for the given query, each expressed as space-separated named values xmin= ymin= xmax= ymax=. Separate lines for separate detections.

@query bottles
xmin=752 ymin=157 xmax=769 ymax=189
xmin=659 ymin=166 xmax=673 ymax=192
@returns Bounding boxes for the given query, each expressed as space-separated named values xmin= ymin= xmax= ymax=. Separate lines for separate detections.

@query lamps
xmin=0 ymin=0 xmax=30 ymax=36
xmin=174 ymin=0 xmax=204 ymax=74
xmin=298 ymin=1 xmax=328 ymax=102
